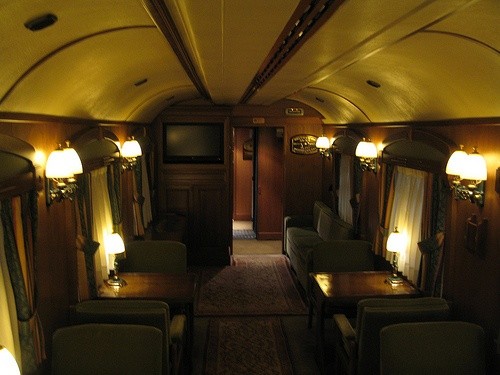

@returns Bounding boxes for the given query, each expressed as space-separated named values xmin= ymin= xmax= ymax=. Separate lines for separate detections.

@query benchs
xmin=283 ymin=201 xmax=353 ymax=304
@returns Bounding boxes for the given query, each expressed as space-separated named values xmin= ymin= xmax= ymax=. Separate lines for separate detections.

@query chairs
xmin=332 ymin=296 xmax=453 ymax=375
xmin=69 ymin=299 xmax=187 ymax=375
xmin=49 ymin=324 xmax=164 ymax=374
xmin=379 ymin=321 xmax=487 ymax=375
xmin=118 ymin=241 xmax=188 ymax=274
xmin=305 ymin=239 xmax=373 ymax=354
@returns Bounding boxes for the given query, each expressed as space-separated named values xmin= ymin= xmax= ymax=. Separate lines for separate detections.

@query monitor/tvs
xmin=162 ymin=122 xmax=224 ymax=164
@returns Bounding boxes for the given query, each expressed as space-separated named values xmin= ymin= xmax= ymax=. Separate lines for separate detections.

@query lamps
xmin=316 ymin=134 xmax=334 ymax=159
xmin=120 ymin=136 xmax=142 ymax=173
xmin=384 ymin=226 xmax=407 ymax=287
xmin=44 ymin=140 xmax=83 ymax=207
xmin=105 ymin=232 xmax=126 ymax=285
xmin=0 ymin=345 xmax=21 ymax=375
xmin=354 ymin=137 xmax=378 ymax=175
xmin=445 ymin=144 xmax=487 ymax=211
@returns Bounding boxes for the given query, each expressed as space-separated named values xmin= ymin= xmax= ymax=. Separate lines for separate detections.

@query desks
xmin=97 ymin=272 xmax=196 ymax=375
xmin=308 ymin=272 xmax=416 ymax=375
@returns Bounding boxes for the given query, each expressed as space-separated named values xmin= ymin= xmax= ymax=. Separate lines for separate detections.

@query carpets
xmin=194 ymin=254 xmax=310 ymax=318
xmin=204 ymin=315 xmax=297 ymax=375
xmin=233 ymin=230 xmax=256 ymax=240
